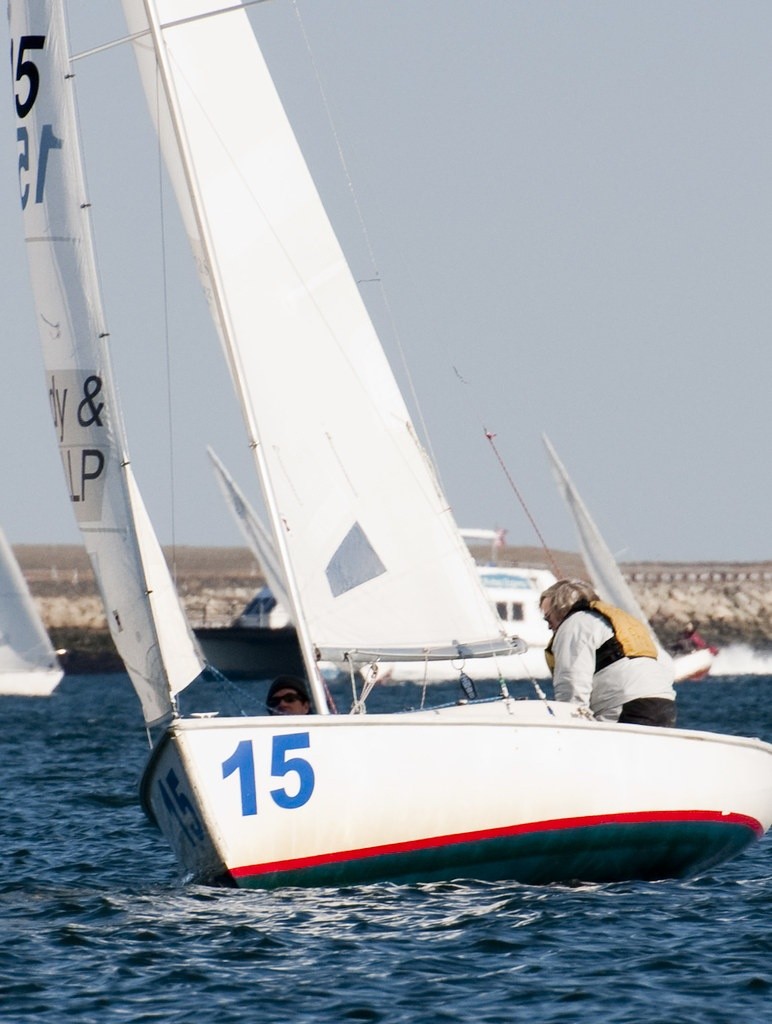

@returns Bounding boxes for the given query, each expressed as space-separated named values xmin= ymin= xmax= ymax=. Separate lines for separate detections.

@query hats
xmin=266 ymin=675 xmax=309 ymax=715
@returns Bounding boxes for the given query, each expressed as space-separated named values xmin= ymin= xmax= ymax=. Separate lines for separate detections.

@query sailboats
xmin=0 ymin=2 xmax=772 ymax=892
xmin=534 ymin=427 xmax=720 ymax=686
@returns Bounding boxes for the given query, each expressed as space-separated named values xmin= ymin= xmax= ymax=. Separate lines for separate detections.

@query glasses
xmin=543 ymin=606 xmax=556 ymax=622
xmin=267 ymin=693 xmax=302 ymax=707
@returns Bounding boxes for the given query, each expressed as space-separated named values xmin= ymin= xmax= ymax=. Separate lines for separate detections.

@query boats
xmin=202 ymin=442 xmax=563 ymax=683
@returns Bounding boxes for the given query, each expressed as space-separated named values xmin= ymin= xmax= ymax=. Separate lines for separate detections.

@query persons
xmin=266 ymin=677 xmax=311 ymax=715
xmin=677 ymin=622 xmax=708 ymax=655
xmin=538 ymin=578 xmax=678 ymax=729
